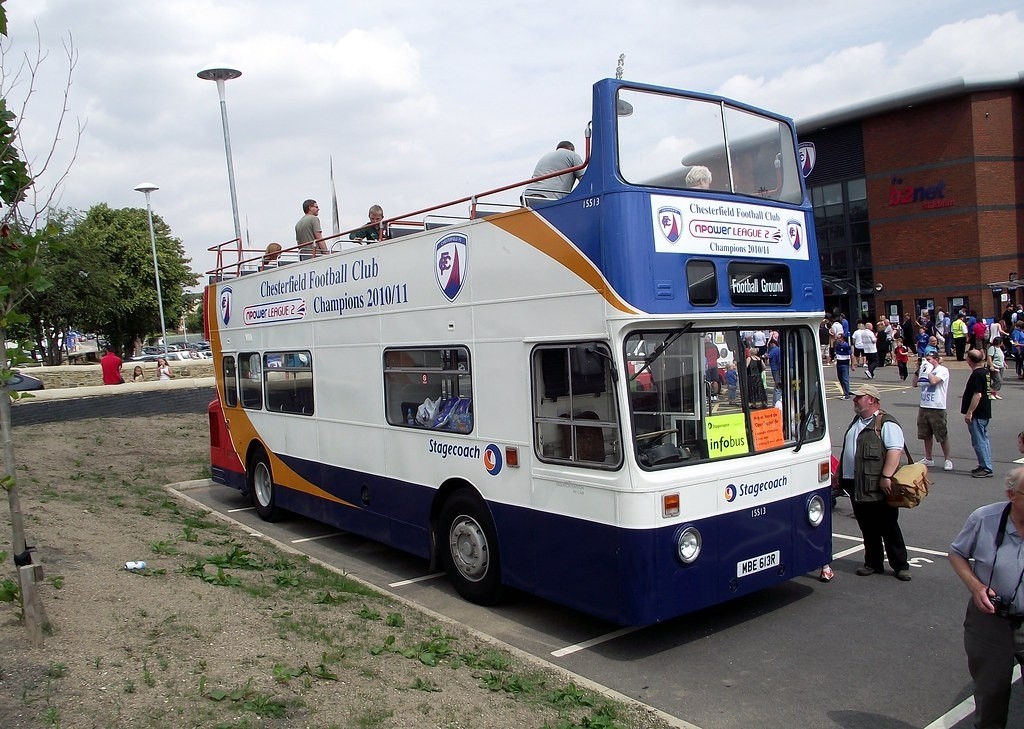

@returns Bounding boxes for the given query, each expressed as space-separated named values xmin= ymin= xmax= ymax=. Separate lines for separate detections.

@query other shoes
xmin=863 ymin=363 xmax=868 ymax=368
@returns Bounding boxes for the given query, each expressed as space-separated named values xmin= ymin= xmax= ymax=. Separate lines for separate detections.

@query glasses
xmin=311 ymin=206 xmax=318 ymax=208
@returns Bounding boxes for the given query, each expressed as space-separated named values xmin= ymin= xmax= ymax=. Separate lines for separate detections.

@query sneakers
xmin=944 ymin=460 xmax=953 ymax=470
xmin=971 ymin=466 xmax=982 ymax=473
xmin=972 ymin=469 xmax=994 ymax=478
xmin=840 ymin=395 xmax=850 ymax=400
xmin=995 ymin=394 xmax=1002 ymax=400
xmin=990 ymin=395 xmax=997 ymax=400
xmin=894 ymin=569 xmax=912 ymax=581
xmin=821 ymin=569 xmax=835 ymax=581
xmin=857 ymin=565 xmax=885 ymax=576
xmin=914 ymin=457 xmax=935 ymax=467
xmin=864 ymin=370 xmax=873 ymax=379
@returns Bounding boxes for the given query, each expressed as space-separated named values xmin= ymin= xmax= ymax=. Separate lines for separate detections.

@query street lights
xmin=196 ymin=68 xmax=246 ymax=270
xmin=133 ymin=183 xmax=170 ymax=362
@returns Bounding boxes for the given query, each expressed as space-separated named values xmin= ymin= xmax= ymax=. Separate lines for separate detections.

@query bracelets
xmin=915 ymin=371 xmax=919 ymax=376
xmin=882 ymin=475 xmax=891 ymax=479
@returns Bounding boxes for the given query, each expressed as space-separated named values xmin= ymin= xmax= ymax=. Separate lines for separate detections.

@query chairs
xmin=562 ymin=411 xmax=606 ymax=463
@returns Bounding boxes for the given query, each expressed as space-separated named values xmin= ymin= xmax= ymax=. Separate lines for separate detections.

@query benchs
xmin=219 ymin=188 xmax=569 ymax=279
xmin=279 ymin=400 xmax=314 ymax=414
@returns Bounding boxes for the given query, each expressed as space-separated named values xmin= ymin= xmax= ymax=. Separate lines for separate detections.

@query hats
xmin=848 ymin=384 xmax=881 ymax=401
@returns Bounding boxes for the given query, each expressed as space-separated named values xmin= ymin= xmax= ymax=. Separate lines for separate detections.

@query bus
xmin=210 ymin=78 xmax=837 ymax=625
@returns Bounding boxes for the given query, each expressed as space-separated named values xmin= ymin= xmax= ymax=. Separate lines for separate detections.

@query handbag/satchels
xmin=887 ymin=463 xmax=935 ymax=509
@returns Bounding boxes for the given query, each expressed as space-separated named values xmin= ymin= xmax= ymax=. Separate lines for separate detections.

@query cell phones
xmin=918 ymin=353 xmax=924 ymax=362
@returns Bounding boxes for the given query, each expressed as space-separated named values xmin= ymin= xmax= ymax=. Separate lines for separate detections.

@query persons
xmin=686 ymin=166 xmax=712 ymax=189
xmin=820 ymin=565 xmax=834 ymax=582
xmin=836 ymin=333 xmax=855 ymax=399
xmin=295 ymin=200 xmax=329 ymax=260
xmin=916 ymin=303 xmax=1024 ymax=380
xmin=704 ymin=330 xmax=782 ymax=409
xmin=101 ymin=346 xmax=125 ymax=385
xmin=130 ymin=366 xmax=143 ymax=382
xmin=157 ymin=358 xmax=173 ymax=380
xmin=520 ymin=141 xmax=586 ymax=204
xmin=912 ymin=351 xmax=952 ymax=470
xmin=961 ymin=350 xmax=993 ymax=478
xmin=349 ymin=205 xmax=392 ymax=245
xmin=264 ymin=243 xmax=282 ymax=265
xmin=819 ymin=313 xmax=849 ymax=365
xmin=987 ymin=337 xmax=1008 ymax=400
xmin=949 ymin=468 xmax=1024 ymax=729
xmin=852 ymin=312 xmax=918 ymax=381
xmin=832 ymin=384 xmax=912 ymax=581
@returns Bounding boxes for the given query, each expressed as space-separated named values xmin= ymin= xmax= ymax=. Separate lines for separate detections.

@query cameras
xmin=986 ymin=595 xmax=1010 ymax=617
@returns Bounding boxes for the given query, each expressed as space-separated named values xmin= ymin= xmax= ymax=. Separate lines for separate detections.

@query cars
xmin=122 ymin=340 xmax=213 ymax=363
xmin=22 ymin=330 xmax=109 ymax=363
